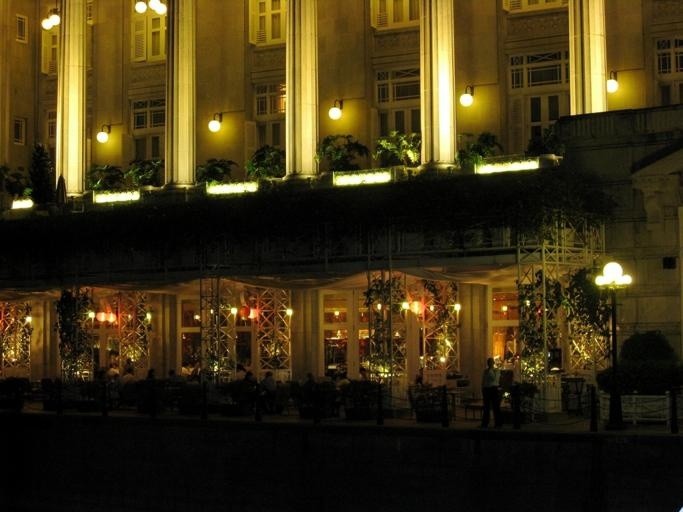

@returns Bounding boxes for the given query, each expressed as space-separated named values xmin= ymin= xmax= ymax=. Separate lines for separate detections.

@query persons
xmin=146 ymin=369 xmax=156 ymax=381
xmin=334 ymin=366 xmax=368 ymax=408
xmin=169 ymin=360 xmax=198 ymax=380
xmin=479 ymin=358 xmax=502 ymax=428
xmin=415 ymin=368 xmax=424 ymax=385
xmin=233 ymin=365 xmax=316 ymax=408
xmin=106 ymin=357 xmax=135 ymax=385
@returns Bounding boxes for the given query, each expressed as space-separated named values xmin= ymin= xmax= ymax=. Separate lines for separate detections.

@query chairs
xmin=0 ymin=372 xmax=585 ymax=425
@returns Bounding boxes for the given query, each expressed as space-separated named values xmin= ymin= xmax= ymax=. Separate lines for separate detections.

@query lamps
xmin=95 ymin=123 xmax=110 ymax=142
xmin=458 ymin=84 xmax=473 ymax=106
xmin=606 ymin=69 xmax=618 ymax=92
xmin=206 ymin=112 xmax=223 ymax=132
xmin=327 ymin=98 xmax=343 ymax=119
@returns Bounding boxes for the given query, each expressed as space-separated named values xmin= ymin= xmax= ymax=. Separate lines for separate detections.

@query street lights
xmin=589 ymin=258 xmax=636 ymax=431
